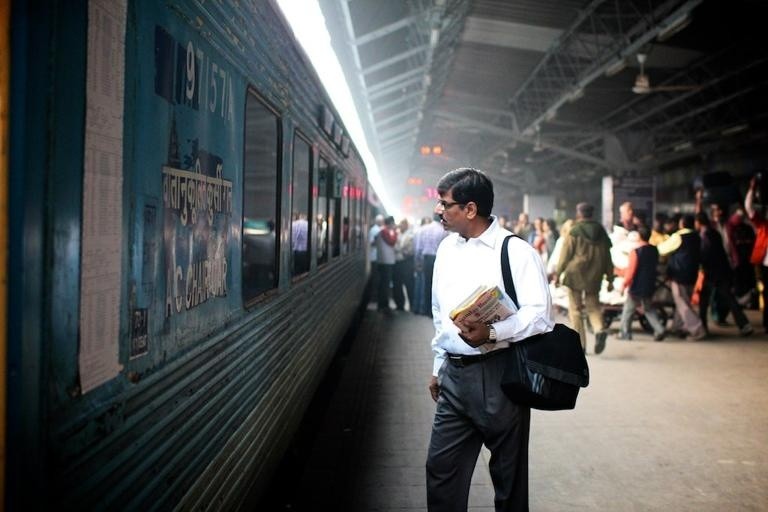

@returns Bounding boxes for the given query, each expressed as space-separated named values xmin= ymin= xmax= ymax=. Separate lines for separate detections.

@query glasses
xmin=437 ymin=199 xmax=466 ymax=208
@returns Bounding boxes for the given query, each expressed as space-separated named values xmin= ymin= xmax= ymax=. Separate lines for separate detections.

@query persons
xmin=366 ymin=199 xmax=768 ymax=359
xmin=243 ymin=209 xmax=368 ymax=286
xmin=424 ymin=165 xmax=559 ymax=511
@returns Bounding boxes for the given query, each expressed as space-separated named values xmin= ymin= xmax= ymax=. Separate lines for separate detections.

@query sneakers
xmin=615 ymin=318 xmax=757 ymax=344
xmin=593 ymin=330 xmax=609 ymax=355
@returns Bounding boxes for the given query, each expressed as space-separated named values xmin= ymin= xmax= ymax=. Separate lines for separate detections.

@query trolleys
xmin=581 ymin=300 xmax=668 ymax=335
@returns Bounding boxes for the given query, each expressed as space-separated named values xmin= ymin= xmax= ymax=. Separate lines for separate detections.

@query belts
xmin=441 ymin=344 xmax=512 ymax=369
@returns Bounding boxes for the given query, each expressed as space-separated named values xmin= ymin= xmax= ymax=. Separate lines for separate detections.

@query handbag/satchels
xmin=497 ymin=231 xmax=592 ymax=414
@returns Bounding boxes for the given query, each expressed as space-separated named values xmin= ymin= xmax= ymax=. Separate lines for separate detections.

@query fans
xmin=582 ymin=47 xmax=698 ymax=95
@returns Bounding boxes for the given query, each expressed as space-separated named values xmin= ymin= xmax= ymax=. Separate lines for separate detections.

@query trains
xmin=1 ymin=0 xmax=387 ymax=512
